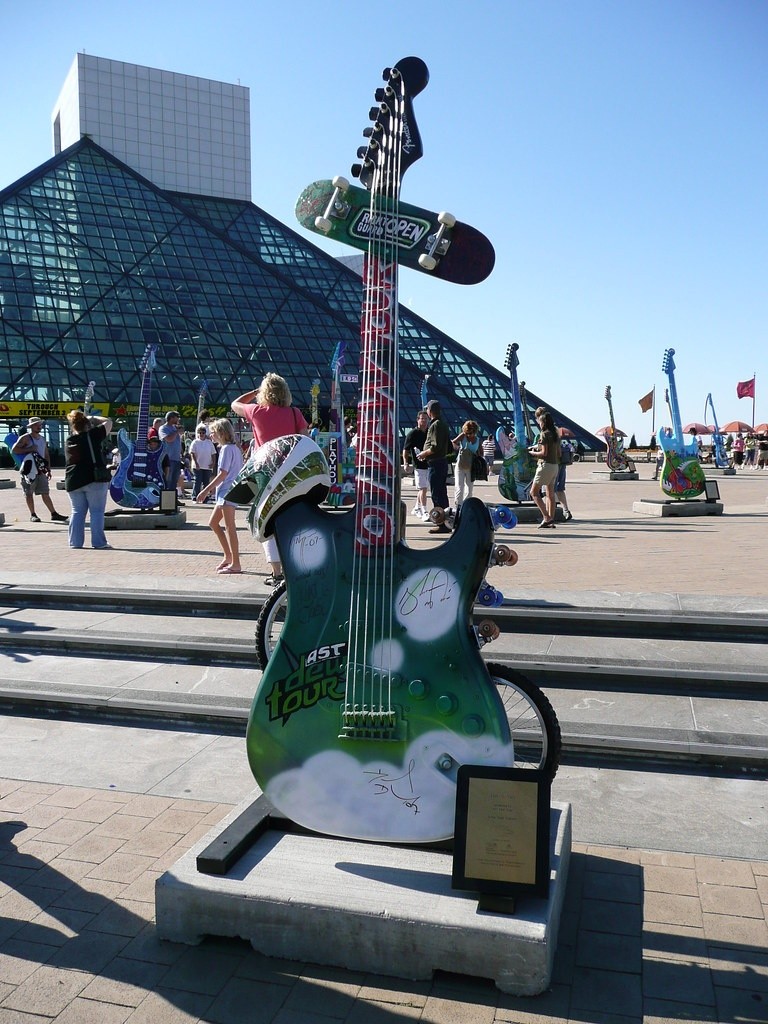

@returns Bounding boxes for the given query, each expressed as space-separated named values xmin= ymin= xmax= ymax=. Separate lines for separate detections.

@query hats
xmin=26 ymin=417 xmax=45 ymax=427
xmin=147 ymin=435 xmax=163 ymax=444
xmin=423 ymin=400 xmax=441 ymax=411
xmin=196 ymin=426 xmax=206 ymax=433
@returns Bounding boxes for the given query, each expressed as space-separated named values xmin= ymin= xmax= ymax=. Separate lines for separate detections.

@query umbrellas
xmin=683 ymin=421 xmax=768 ymax=446
xmin=557 ymin=426 xmax=575 ymax=438
xmin=596 ymin=426 xmax=628 ymax=437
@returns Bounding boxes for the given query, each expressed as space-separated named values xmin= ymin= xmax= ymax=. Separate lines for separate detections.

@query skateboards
xmin=295 ymin=175 xmax=496 ymax=284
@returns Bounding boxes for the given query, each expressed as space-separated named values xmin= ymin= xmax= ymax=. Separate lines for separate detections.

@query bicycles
xmin=254 ymin=571 xmax=562 ymax=788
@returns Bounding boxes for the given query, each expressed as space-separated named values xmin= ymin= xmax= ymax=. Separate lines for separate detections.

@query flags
xmin=638 ymin=389 xmax=653 ymax=413
xmin=737 ymin=378 xmax=755 ymax=399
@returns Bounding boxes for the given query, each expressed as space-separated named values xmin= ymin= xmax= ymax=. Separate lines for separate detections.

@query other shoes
xmin=29 ymin=515 xmax=41 ymax=522
xmin=264 ymin=572 xmax=284 ymax=587
xmin=538 ymin=519 xmax=557 ymax=528
xmin=421 ymin=513 xmax=432 ymax=521
xmin=565 ymin=510 xmax=572 ymax=521
xmin=428 ymin=525 xmax=452 ymax=533
xmin=651 ymin=476 xmax=658 ymax=480
xmin=176 ymin=489 xmax=215 ymax=508
xmin=411 ymin=508 xmax=423 ymax=520
xmin=94 ymin=543 xmax=113 ymax=549
xmin=489 ymin=471 xmax=495 ymax=476
xmin=51 ymin=514 xmax=69 ymax=521
xmin=726 ymin=463 xmax=768 ymax=472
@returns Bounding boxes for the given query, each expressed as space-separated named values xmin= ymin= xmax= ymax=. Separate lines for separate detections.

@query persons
xmin=652 ymin=428 xmax=673 ymax=480
xmin=12 ymin=417 xmax=69 ymax=522
xmin=231 ymin=373 xmax=308 ymax=588
xmin=689 ymin=427 xmax=703 ymax=464
xmin=482 ymin=434 xmax=495 ymax=476
xmin=528 ymin=407 xmax=572 ymax=529
xmin=307 ymin=423 xmax=318 ymax=441
xmin=196 ymin=417 xmax=243 ymax=575
xmin=344 ymin=416 xmax=358 ymax=447
xmin=145 ymin=411 xmax=251 ymax=504
xmin=111 ymin=448 xmax=121 ymax=466
xmin=65 ymin=410 xmax=112 ymax=549
xmin=403 ymin=399 xmax=483 ymax=534
xmin=561 ymin=440 xmax=577 ymax=453
xmin=724 ymin=430 xmax=768 ymax=471
xmin=504 ymin=430 xmax=517 ymax=445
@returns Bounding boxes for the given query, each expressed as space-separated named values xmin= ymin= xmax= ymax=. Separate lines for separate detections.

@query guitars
xmin=420 ymin=374 xmax=432 ymax=428
xmin=495 ymin=342 xmax=550 ymax=502
xmin=110 ymin=343 xmax=169 ymax=509
xmin=602 ymin=385 xmax=628 ymax=470
xmin=84 ymin=380 xmax=96 ymax=427
xmin=658 ymin=347 xmax=707 ymax=499
xmin=307 ymin=340 xmax=355 ymax=507
xmin=183 ymin=378 xmax=212 ymax=481
xmin=707 ymin=393 xmax=730 ymax=466
xmin=243 ymin=57 xmax=514 ymax=846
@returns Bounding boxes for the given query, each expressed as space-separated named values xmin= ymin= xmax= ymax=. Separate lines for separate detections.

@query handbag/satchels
xmin=19 ymin=452 xmax=51 ymax=486
xmin=93 ymin=462 xmax=111 ymax=481
xmin=457 ymin=447 xmax=474 ymax=469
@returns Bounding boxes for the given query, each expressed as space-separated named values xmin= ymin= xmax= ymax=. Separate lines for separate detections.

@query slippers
xmin=215 ymin=561 xmax=243 ymax=574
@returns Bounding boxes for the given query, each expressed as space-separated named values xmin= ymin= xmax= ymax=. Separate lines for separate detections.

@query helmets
xmin=223 ymin=434 xmax=332 ymax=544
